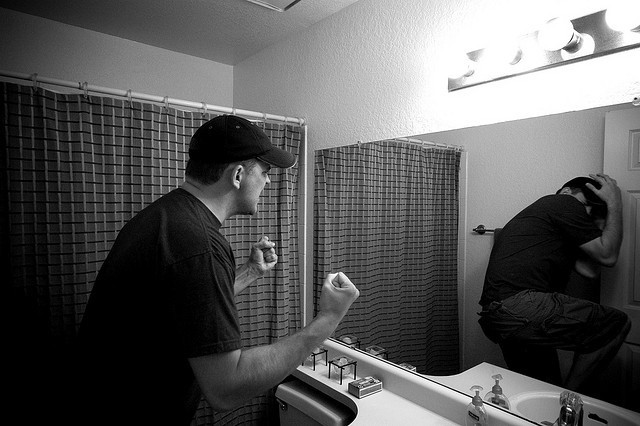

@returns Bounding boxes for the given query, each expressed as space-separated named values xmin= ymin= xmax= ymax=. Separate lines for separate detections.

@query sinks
xmin=508 ymin=393 xmax=640 ymax=426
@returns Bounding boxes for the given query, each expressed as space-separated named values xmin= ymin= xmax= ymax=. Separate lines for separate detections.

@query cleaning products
xmin=465 ymin=384 xmax=487 ymax=426
xmin=482 ymin=375 xmax=510 ymax=408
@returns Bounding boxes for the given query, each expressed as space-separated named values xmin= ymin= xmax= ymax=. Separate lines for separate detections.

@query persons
xmin=77 ymin=115 xmax=360 ymax=425
xmin=476 ymin=173 xmax=632 ymax=390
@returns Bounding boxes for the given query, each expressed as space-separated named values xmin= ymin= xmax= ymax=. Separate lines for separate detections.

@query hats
xmin=188 ymin=114 xmax=298 ymax=170
xmin=561 ymin=177 xmax=608 ymax=220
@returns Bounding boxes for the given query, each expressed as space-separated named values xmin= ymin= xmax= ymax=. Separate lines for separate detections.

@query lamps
xmin=536 ymin=14 xmax=585 ymax=55
xmin=561 ymin=32 xmax=595 ymax=61
xmin=605 ymin=11 xmax=640 ymax=38
xmin=440 ymin=50 xmax=477 ymax=81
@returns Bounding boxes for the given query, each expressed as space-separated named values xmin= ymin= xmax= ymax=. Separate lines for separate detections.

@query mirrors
xmin=312 ymin=106 xmax=640 ymax=426
